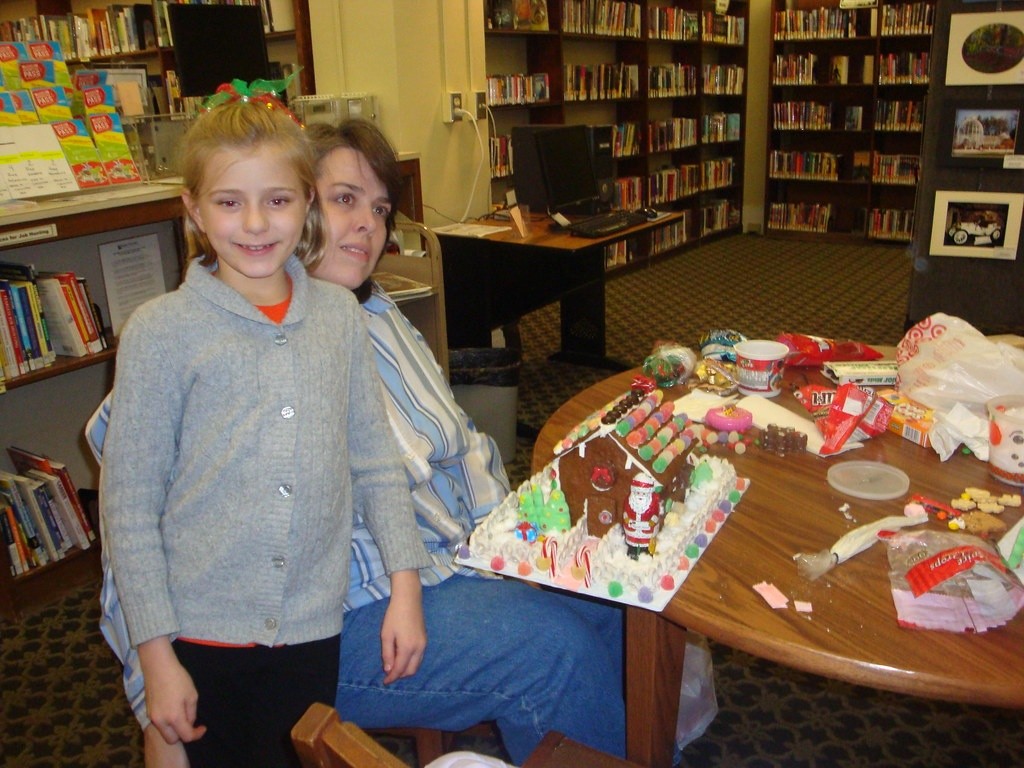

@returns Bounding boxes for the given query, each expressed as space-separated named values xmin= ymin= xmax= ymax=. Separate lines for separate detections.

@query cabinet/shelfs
xmin=34 ymin=0 xmax=318 ymax=117
xmin=481 ymin=1 xmax=935 ymax=278
xmin=0 ymin=157 xmax=426 ymax=621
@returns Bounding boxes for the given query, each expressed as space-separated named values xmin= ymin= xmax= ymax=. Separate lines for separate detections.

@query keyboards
xmin=559 ymin=208 xmax=647 ymax=239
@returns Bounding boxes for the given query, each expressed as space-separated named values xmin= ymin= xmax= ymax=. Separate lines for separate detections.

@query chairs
xmin=289 ymin=702 xmax=637 ymax=767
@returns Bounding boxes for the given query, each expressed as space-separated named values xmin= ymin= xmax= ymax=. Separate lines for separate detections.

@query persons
xmin=98 ymin=67 xmax=437 ymax=768
xmin=86 ymin=118 xmax=681 ymax=768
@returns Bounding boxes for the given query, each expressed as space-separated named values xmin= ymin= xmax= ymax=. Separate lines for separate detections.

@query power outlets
xmin=440 ymin=91 xmax=462 ymax=123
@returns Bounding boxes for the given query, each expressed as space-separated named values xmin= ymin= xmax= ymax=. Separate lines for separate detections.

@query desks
xmin=531 ymin=342 xmax=1024 ymax=768
xmin=447 ymin=205 xmax=682 ymax=359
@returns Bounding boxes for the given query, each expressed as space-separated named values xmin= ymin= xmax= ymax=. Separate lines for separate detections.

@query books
xmin=0 ymin=0 xmax=296 ymax=58
xmin=0 ymin=446 xmax=97 ymax=584
xmin=0 ymin=262 xmax=107 ymax=387
xmin=142 ymin=70 xmax=208 ymax=122
xmin=0 ymin=223 xmax=59 ymax=247
xmin=0 ymin=43 xmax=141 ymax=204
xmin=767 ymin=2 xmax=936 ymax=248
xmin=483 ymin=0 xmax=747 ymax=267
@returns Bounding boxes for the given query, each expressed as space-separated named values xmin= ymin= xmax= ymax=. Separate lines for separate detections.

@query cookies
xmin=947 ymin=486 xmax=1021 ymax=536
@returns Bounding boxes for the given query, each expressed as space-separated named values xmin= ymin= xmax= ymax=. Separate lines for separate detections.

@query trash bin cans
xmin=448 ymin=348 xmax=521 ymax=464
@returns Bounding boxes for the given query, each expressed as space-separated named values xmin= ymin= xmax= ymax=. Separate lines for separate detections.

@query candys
xmin=938 ymin=492 xmax=971 ymax=531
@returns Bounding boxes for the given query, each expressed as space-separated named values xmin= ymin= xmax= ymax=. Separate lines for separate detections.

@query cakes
xmin=456 ymin=376 xmax=745 ymax=605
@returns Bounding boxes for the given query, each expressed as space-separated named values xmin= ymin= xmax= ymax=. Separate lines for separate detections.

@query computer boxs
xmin=511 ymin=125 xmax=615 ymax=216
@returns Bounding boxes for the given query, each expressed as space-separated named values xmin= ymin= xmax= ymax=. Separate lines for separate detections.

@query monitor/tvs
xmin=533 ymin=124 xmax=600 ymax=230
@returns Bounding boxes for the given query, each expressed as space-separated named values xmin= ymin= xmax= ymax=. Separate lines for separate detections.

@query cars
xmin=947 ymin=221 xmax=1002 ymax=246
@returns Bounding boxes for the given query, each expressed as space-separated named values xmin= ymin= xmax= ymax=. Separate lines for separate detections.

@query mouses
xmin=635 ymin=208 xmax=657 ymax=218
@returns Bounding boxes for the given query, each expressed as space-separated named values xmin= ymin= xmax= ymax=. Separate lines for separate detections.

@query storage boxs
xmin=875 ymin=389 xmax=933 ymax=449
xmin=819 ymin=361 xmax=900 ymax=384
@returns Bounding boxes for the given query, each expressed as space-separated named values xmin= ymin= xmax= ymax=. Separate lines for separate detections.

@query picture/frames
xmin=929 ymin=190 xmax=1024 ymax=261
xmin=936 ymin=97 xmax=1024 ymax=173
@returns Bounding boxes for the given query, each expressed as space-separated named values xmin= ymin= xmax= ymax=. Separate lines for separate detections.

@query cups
xmin=987 ymin=394 xmax=1024 ymax=487
xmin=734 ymin=339 xmax=789 ymax=398
